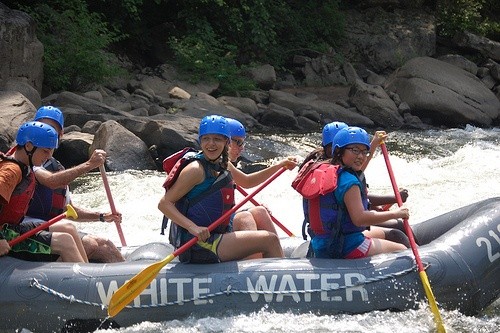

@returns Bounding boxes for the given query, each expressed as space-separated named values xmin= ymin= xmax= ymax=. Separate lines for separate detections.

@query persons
xmin=304 ymin=121 xmax=420 ymax=245
xmin=0 ymin=105 xmax=124 ymax=262
xmin=308 ymin=126 xmax=410 ymax=258
xmin=158 ymin=115 xmax=298 ymax=263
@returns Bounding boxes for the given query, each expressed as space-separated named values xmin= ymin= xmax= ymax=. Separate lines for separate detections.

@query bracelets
xmin=100 ymin=213 xmax=104 ymax=222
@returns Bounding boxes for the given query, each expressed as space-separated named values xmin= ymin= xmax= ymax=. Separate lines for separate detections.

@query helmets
xmin=34 ymin=106 xmax=64 ymax=130
xmin=226 ymin=118 xmax=248 ymax=137
xmin=321 ymin=121 xmax=349 ymax=146
xmin=198 ymin=114 xmax=231 ymax=140
xmin=16 ymin=121 xmax=58 ymax=150
xmin=331 ymin=127 xmax=371 ymax=159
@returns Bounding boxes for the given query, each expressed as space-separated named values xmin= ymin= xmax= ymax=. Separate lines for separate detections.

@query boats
xmin=0 ymin=193 xmax=500 ymax=333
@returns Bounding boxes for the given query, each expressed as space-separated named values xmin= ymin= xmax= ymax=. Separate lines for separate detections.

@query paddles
xmin=6 ymin=204 xmax=78 ymax=246
xmin=100 ymin=164 xmax=127 ymax=247
xmin=382 ymin=188 xmax=404 ymax=211
xmin=233 ymin=183 xmax=295 ymax=237
xmin=379 ymin=134 xmax=446 ymax=333
xmin=108 ymin=167 xmax=288 ymax=318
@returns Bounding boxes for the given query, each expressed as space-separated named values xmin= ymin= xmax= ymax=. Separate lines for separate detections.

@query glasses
xmin=232 ymin=138 xmax=247 ymax=147
xmin=346 ymin=147 xmax=371 ymax=157
